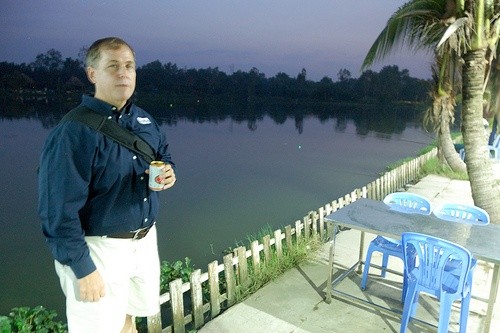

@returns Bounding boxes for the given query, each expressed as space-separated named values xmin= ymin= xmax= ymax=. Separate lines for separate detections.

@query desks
xmin=322 ymin=197 xmax=500 ymax=333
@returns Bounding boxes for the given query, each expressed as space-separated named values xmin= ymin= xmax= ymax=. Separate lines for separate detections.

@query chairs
xmin=361 ymin=192 xmax=490 ymax=333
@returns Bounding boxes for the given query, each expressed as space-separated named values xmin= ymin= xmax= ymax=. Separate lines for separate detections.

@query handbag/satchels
xmin=67 ymin=105 xmax=156 ymax=164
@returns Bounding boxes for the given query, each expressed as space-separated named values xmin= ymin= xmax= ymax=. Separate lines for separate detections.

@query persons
xmin=38 ymin=38 xmax=176 ymax=333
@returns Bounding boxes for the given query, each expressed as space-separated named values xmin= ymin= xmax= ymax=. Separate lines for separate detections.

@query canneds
xmin=148 ymin=161 xmax=165 ymax=191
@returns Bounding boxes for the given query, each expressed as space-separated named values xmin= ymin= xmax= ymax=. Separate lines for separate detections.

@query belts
xmin=109 ymin=225 xmax=153 ymax=239
